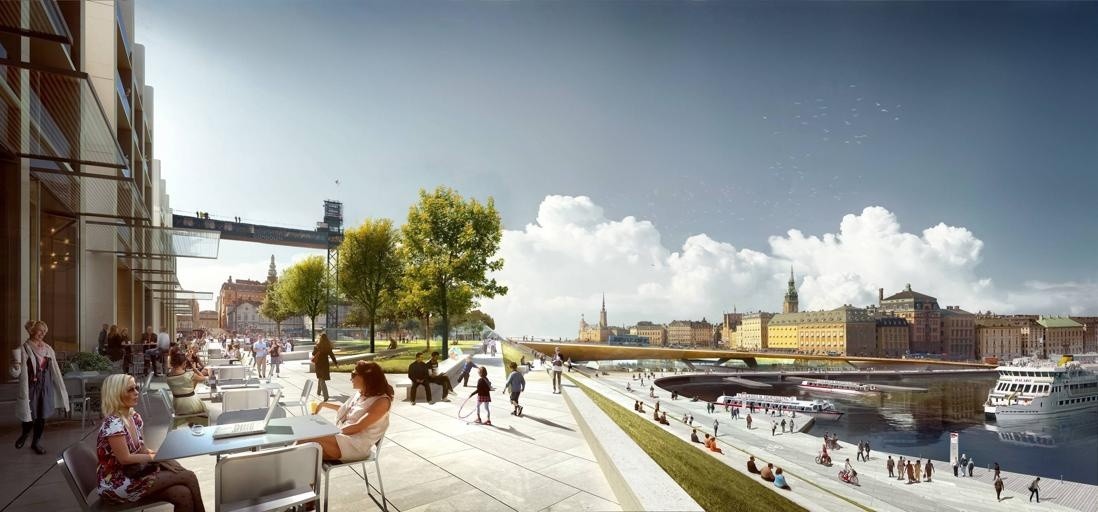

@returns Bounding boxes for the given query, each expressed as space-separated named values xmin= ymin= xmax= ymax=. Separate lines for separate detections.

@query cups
xmin=192 ymin=424 xmax=204 ymax=435
xmin=308 ymin=399 xmax=317 ymax=421
xmin=11 ymin=348 xmax=22 ymax=365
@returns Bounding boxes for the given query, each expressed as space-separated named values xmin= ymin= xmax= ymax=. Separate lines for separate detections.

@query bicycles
xmin=814 ymin=450 xmax=830 ymax=467
xmin=837 ymin=470 xmax=858 ymax=486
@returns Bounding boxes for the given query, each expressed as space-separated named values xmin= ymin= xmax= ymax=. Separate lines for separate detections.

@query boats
xmin=982 ymin=409 xmax=1097 ymax=450
xmin=712 ymin=391 xmax=844 ymax=423
xmin=982 ymin=351 xmax=1098 ymax=422
xmin=799 ymin=379 xmax=883 ymax=397
xmin=804 ymin=389 xmax=869 ymax=402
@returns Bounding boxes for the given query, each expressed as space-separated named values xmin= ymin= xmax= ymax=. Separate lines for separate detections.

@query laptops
xmin=213 ymin=389 xmax=282 ymax=439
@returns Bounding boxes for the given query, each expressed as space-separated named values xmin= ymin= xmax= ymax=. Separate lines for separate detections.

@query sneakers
xmin=552 ymin=390 xmax=562 ymax=394
xmin=474 ymin=419 xmax=492 ymax=426
xmin=511 ymin=410 xmax=517 ymax=416
xmin=409 ymin=390 xmax=457 ymax=406
xmin=517 ymin=405 xmax=524 ymax=416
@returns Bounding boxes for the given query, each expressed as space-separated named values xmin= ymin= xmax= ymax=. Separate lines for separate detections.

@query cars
xmin=829 ymin=352 xmax=841 ymax=356
xmin=669 ymin=344 xmax=685 ymax=349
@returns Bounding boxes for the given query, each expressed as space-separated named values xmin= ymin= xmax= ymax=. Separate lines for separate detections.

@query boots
xmin=14 ymin=430 xmax=28 ymax=449
xmin=30 ymin=437 xmax=48 ymax=455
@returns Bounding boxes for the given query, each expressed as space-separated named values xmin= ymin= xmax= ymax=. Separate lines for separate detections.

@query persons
xmin=94 ymin=316 xmax=574 ymax=479
xmin=8 ymin=316 xmax=68 ymax=453
xmin=592 ymin=361 xmax=977 ymax=491
xmin=989 ymin=463 xmax=1047 ymax=503
xmin=92 ymin=375 xmax=206 ymax=512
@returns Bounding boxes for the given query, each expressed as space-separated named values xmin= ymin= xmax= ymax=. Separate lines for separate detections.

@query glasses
xmin=126 ymin=385 xmax=140 ymax=393
xmin=350 ymin=372 xmax=360 ymax=378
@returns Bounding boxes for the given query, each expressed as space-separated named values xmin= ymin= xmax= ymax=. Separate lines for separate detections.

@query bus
xmin=608 ymin=334 xmax=650 ymax=347
xmin=282 ymin=327 xmax=306 ymax=338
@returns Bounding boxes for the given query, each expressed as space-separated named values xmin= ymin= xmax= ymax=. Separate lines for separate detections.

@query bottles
xmin=211 ymin=370 xmax=216 ymax=389
